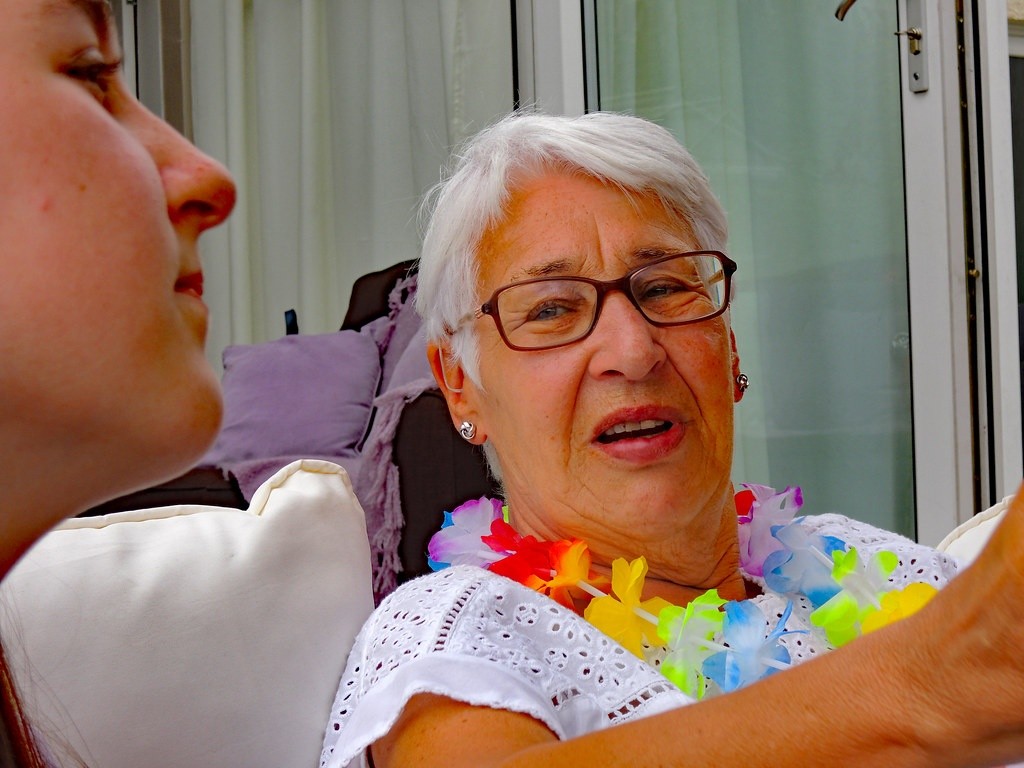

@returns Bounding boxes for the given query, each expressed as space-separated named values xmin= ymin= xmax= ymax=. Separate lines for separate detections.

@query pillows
xmin=199 ymin=330 xmax=380 ymax=467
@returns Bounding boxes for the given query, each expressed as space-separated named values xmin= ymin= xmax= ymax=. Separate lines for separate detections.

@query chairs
xmin=124 ymin=258 xmax=488 ymax=601
xmin=0 ymin=459 xmax=374 ymax=768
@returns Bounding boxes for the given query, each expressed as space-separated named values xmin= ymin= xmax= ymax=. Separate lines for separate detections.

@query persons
xmin=344 ymin=109 xmax=1024 ymax=768
xmin=0 ymin=1 xmax=242 ymax=582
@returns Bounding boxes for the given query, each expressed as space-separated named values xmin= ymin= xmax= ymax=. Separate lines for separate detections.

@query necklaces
xmin=424 ymin=476 xmax=939 ymax=697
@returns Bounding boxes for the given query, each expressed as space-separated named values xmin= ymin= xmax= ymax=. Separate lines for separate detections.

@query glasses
xmin=446 ymin=249 xmax=739 ymax=351
